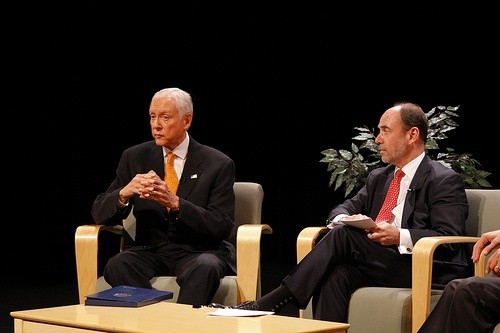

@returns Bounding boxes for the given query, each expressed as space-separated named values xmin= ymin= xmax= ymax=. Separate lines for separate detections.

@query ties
xmin=375 ymin=169 xmax=405 ymax=224
xmin=165 ymin=152 xmax=178 ymax=213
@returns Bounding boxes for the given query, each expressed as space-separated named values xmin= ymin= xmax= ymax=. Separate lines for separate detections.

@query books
xmin=84 ymin=285 xmax=174 ymax=308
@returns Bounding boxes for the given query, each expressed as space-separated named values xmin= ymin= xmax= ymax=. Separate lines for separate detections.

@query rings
xmin=496 ymin=265 xmax=500 ymax=270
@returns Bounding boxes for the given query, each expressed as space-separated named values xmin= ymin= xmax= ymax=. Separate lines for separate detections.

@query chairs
xmin=75 ymin=182 xmax=273 ymax=307
xmin=297 ymin=188 xmax=500 ymax=333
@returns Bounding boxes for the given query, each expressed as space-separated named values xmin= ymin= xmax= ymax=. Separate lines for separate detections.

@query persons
xmin=208 ymin=103 xmax=469 ymax=323
xmin=417 ymin=230 xmax=500 ymax=333
xmin=91 ymin=88 xmax=236 ymax=307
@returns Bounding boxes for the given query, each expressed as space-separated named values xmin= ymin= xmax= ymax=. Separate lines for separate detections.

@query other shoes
xmin=208 ymin=299 xmax=299 ymax=317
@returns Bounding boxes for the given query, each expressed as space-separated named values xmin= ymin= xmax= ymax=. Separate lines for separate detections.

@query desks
xmin=11 ymin=304 xmax=350 ymax=333
